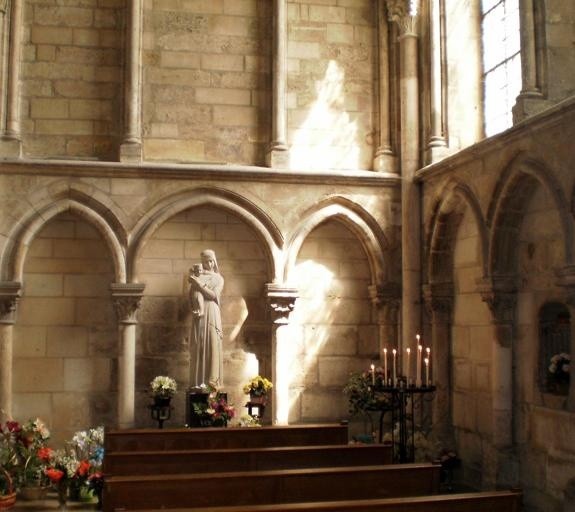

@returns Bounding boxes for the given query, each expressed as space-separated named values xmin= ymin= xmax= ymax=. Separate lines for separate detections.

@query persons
xmin=187 ymin=249 xmax=224 ymax=389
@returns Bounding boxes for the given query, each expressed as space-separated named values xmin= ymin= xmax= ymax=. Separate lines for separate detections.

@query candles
xmin=370 ymin=338 xmax=431 ymax=390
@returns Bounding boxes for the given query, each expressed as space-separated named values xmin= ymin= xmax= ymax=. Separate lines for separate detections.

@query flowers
xmin=1 ymin=416 xmax=105 ymax=500
xmin=244 ymin=375 xmax=274 ymax=400
xmin=192 ymin=396 xmax=236 ymax=427
xmin=344 ymin=369 xmax=412 ymax=415
xmin=151 ymin=376 xmax=176 ymax=400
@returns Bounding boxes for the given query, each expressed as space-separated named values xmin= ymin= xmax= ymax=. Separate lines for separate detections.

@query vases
xmin=251 ymin=393 xmax=264 ymax=405
xmin=155 ymin=394 xmax=172 ymax=405
xmin=63 ymin=494 xmax=99 ymax=512
xmin=0 ymin=492 xmax=16 ymax=512
